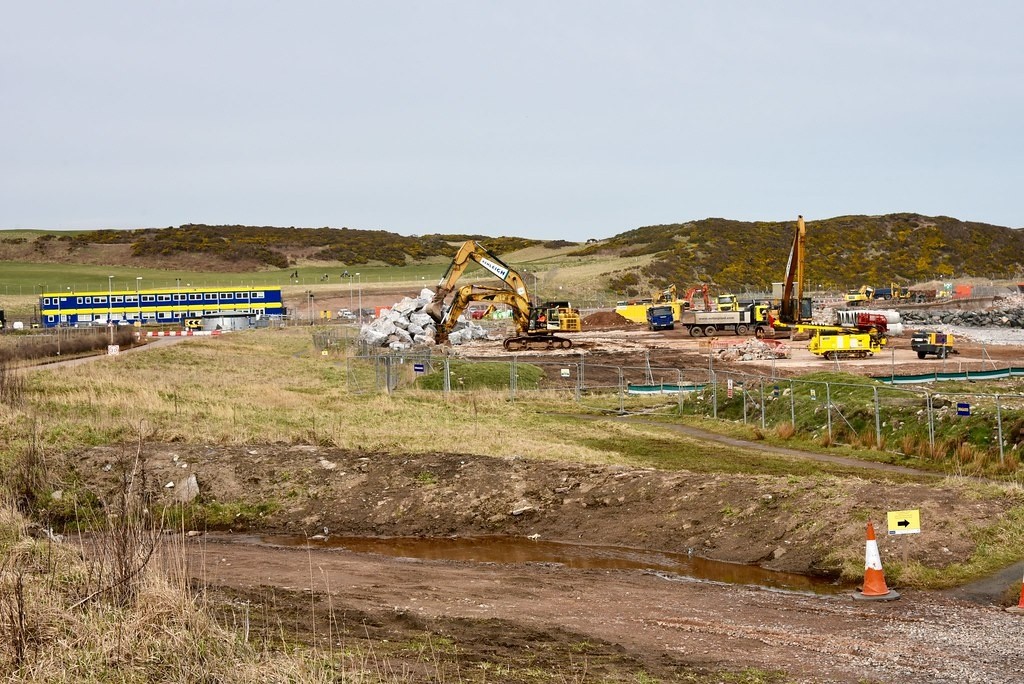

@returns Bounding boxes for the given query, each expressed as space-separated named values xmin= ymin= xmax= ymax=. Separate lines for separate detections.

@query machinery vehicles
xmin=426 ymin=240 xmax=581 ymax=351
xmin=617 ymin=216 xmax=1023 ymax=359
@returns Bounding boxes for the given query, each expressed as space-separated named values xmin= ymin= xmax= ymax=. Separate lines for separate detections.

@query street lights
xmin=356 ymin=272 xmax=362 ymax=324
xmin=136 ymin=276 xmax=143 ymax=321
xmin=108 ymin=275 xmax=116 ymax=322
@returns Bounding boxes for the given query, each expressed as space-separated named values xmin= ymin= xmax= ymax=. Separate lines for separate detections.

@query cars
xmin=338 ymin=307 xmax=376 ymax=322
xmin=118 ymin=319 xmax=130 ymax=325
xmin=146 ymin=318 xmax=158 ymax=324
xmin=91 ymin=318 xmax=107 ymax=326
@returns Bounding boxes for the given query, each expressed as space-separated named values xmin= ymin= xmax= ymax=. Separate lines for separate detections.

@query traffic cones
xmin=1004 ymin=574 xmax=1024 ymax=617
xmin=850 ymin=516 xmax=901 ymax=602
